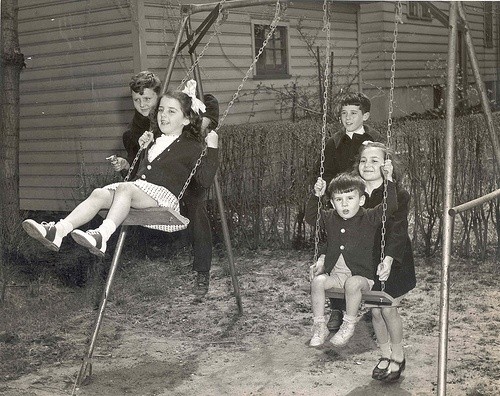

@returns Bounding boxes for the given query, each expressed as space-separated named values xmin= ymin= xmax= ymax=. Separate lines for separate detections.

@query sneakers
xmin=70 ymin=228 xmax=107 ymax=259
xmin=22 ymin=219 xmax=64 ymax=252
xmin=308 ymin=321 xmax=329 ymax=347
xmin=329 ymin=319 xmax=355 ymax=346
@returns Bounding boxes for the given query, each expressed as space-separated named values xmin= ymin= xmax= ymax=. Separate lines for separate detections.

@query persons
xmin=106 ymin=70 xmax=220 ymax=296
xmin=358 ymin=140 xmax=417 ymax=384
xmin=320 ymin=91 xmax=384 ymax=331
xmin=304 ymin=158 xmax=399 ymax=348
xmin=21 ymin=90 xmax=219 ymax=257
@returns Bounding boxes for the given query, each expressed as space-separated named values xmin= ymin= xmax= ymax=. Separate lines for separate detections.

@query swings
xmin=97 ymin=4 xmax=280 ymax=226
xmin=298 ymin=0 xmax=402 ymax=308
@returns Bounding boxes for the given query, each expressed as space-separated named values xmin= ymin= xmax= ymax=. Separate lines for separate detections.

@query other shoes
xmin=327 ymin=311 xmax=344 ymax=330
xmin=192 ymin=270 xmax=210 ymax=296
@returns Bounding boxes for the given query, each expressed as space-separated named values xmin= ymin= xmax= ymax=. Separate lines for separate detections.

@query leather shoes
xmin=372 ymin=356 xmax=391 ymax=380
xmin=383 ymin=358 xmax=406 ymax=382
